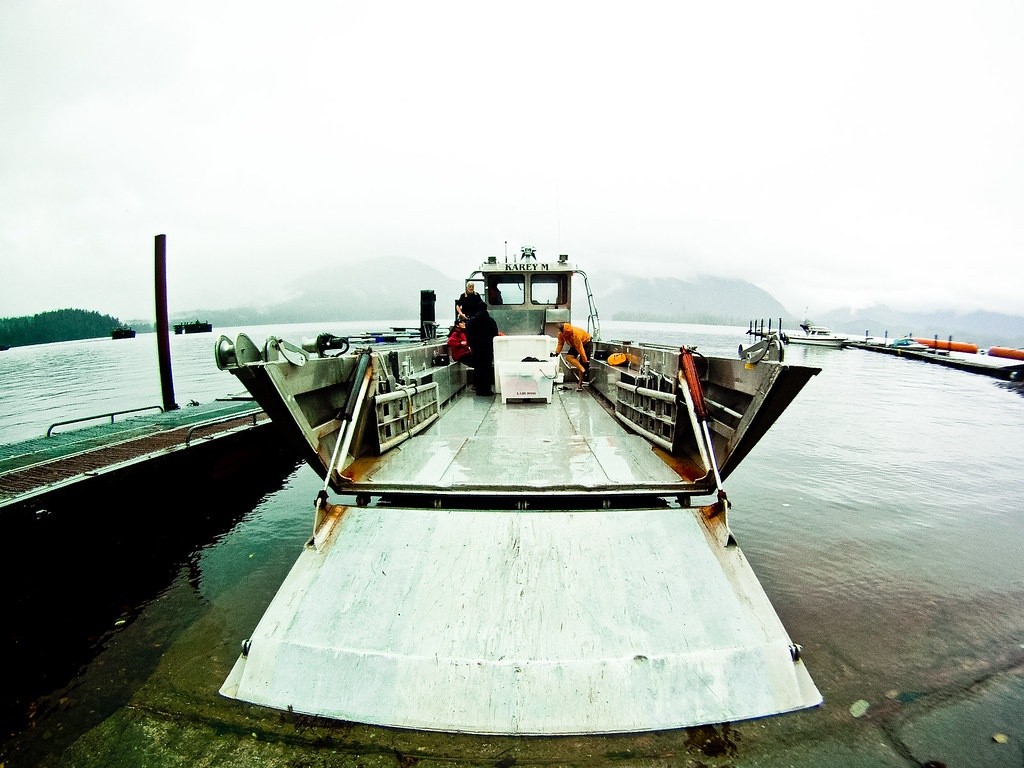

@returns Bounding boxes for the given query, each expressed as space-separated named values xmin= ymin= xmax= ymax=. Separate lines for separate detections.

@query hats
xmin=454 ymin=318 xmax=467 ymax=325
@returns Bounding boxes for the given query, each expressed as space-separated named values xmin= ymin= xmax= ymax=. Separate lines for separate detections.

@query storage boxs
xmin=496 ymin=360 xmax=559 ymax=405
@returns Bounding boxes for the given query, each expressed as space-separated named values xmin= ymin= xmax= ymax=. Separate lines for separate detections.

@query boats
xmin=783 ymin=319 xmax=848 ymax=347
xmin=892 ymin=337 xmax=929 ymax=352
xmin=0 ymin=343 xmax=12 ymax=351
xmin=215 ymin=239 xmax=823 ymax=737
xmin=111 ymin=326 xmax=136 ymax=340
xmin=173 ymin=318 xmax=213 ymax=335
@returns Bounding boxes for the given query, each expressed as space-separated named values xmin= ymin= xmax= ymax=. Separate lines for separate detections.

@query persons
xmin=470 ymin=301 xmax=499 ymax=390
xmin=555 ymin=323 xmax=590 ymax=392
xmin=448 ymin=318 xmax=495 ymax=396
xmin=455 ymin=281 xmax=483 ymax=319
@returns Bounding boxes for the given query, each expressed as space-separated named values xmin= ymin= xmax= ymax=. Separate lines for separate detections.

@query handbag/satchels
xmin=435 ymin=353 xmax=449 ymax=366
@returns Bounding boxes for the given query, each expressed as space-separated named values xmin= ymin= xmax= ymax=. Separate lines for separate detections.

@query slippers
xmin=558 ymin=385 xmax=573 ymax=391
xmin=576 ymin=386 xmax=583 ymax=391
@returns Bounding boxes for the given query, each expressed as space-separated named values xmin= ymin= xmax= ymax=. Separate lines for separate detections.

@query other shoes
xmin=476 ymin=390 xmax=495 ymax=397
